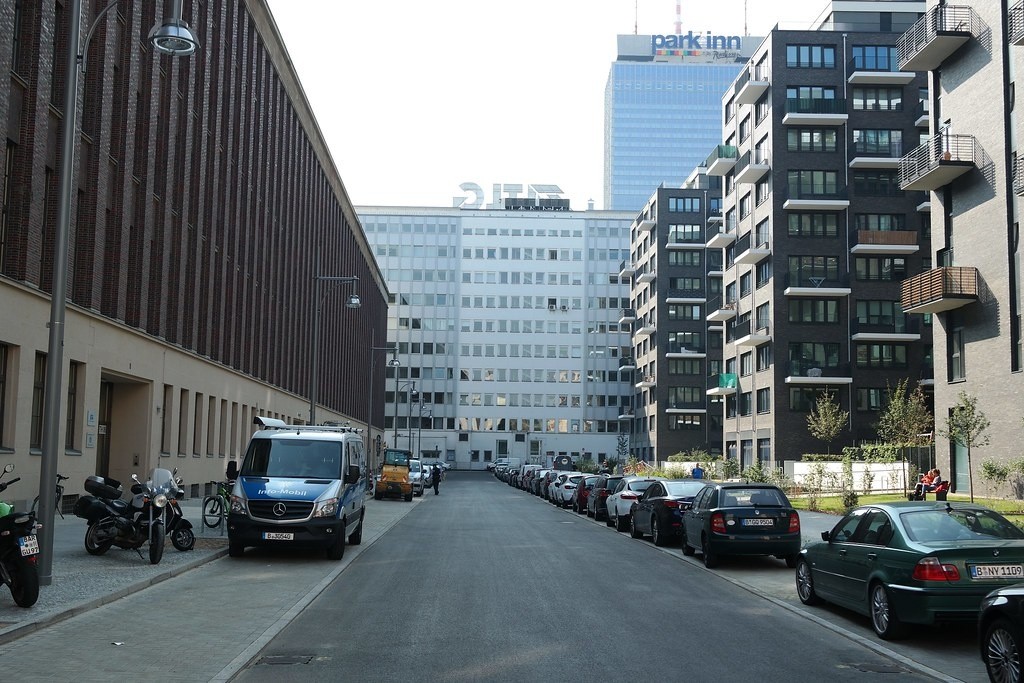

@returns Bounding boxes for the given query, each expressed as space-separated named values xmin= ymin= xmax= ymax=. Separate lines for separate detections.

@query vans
xmin=227 ymin=415 xmax=369 ymax=560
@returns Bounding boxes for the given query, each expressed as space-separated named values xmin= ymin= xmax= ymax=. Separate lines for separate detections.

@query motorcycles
xmin=74 ymin=468 xmax=197 ymax=564
xmin=0 ymin=465 xmax=41 ymax=607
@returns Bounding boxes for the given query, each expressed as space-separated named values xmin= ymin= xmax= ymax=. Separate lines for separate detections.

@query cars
xmin=678 ymin=483 xmax=801 ymax=569
xmin=487 ymin=456 xmax=667 ymax=530
xmin=977 ymin=583 xmax=1023 ymax=682
xmin=628 ymin=479 xmax=710 ymax=546
xmin=792 ymin=499 xmax=1024 ymax=638
xmin=411 ymin=460 xmax=451 ymax=497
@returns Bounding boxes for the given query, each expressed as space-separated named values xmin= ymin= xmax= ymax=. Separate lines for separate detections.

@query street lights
xmin=393 ymin=378 xmax=418 ymax=447
xmin=309 ymin=273 xmax=364 ymax=427
xmin=408 ymin=398 xmax=433 ymax=457
xmin=364 ymin=346 xmax=401 ymax=493
xmin=28 ymin=0 xmax=199 ymax=586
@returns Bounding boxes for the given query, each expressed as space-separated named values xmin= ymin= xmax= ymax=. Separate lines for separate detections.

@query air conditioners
xmin=549 ymin=305 xmax=555 ymax=311
xmin=560 ymin=305 xmax=568 ymax=310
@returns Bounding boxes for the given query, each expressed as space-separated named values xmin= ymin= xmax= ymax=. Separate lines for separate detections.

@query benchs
xmin=926 ymin=482 xmax=953 ymax=501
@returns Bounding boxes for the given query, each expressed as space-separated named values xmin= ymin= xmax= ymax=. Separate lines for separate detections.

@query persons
xmin=912 ymin=468 xmax=941 ymax=496
xmin=432 ymin=464 xmax=441 ymax=495
xmin=692 ymin=463 xmax=703 ymax=479
xmin=572 ymin=461 xmax=578 ymax=471
xmin=597 ymin=459 xmax=612 ymax=474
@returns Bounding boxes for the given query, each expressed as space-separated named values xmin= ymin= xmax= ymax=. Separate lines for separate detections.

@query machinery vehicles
xmin=375 ymin=448 xmax=413 ymax=501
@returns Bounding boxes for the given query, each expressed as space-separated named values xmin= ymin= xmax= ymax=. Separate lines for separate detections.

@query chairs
xmin=874 ymin=525 xmax=890 ymax=542
xmin=725 ymin=496 xmax=738 ymax=507
xmin=751 ymin=494 xmax=777 ymax=504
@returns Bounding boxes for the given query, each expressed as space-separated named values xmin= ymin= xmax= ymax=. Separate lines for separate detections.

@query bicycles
xmin=202 ymin=481 xmax=237 ymax=528
xmin=32 ymin=472 xmax=70 ymax=524
